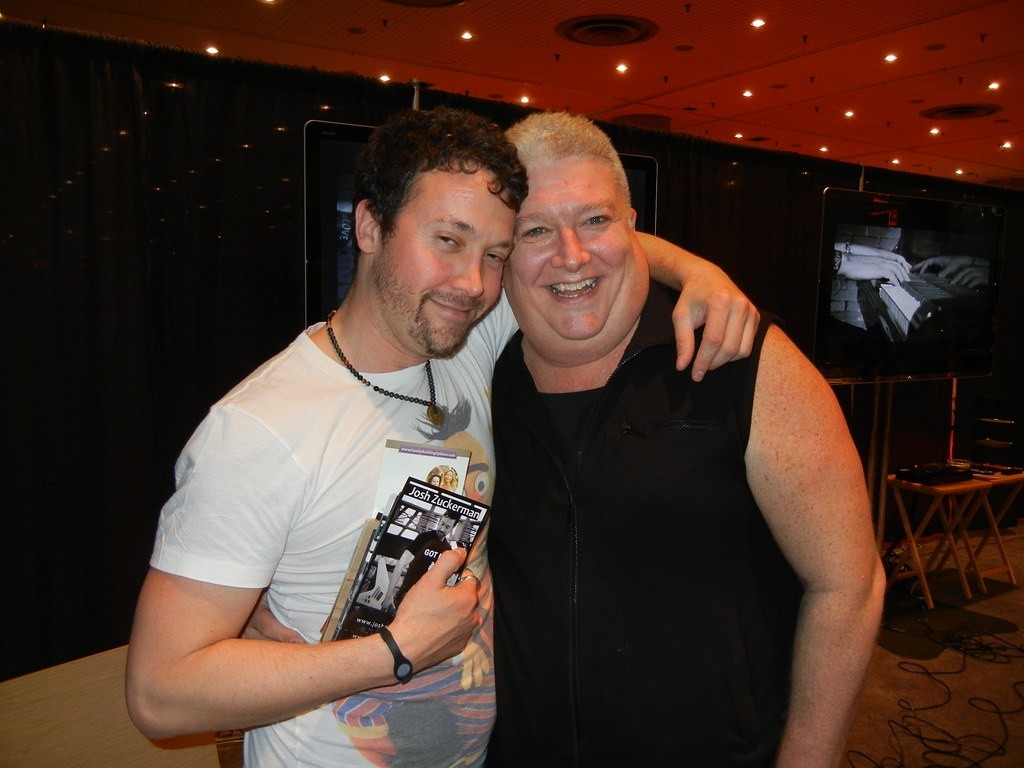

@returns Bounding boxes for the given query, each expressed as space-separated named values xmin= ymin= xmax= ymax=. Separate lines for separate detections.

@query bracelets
xmin=834 ymin=250 xmax=842 ymax=273
xmin=379 ymin=627 xmax=412 ymax=685
xmin=845 ymin=243 xmax=852 ymax=253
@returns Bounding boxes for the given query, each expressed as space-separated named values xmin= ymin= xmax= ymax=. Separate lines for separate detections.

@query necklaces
xmin=328 ymin=310 xmax=446 ymax=424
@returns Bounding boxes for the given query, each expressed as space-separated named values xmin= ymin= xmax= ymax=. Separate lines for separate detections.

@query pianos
xmin=872 ymin=268 xmax=964 ymax=343
xmin=914 ymin=270 xmax=972 ymax=300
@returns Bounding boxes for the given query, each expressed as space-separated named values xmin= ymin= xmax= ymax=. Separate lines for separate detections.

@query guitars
xmin=356 ymin=558 xmax=396 ymax=616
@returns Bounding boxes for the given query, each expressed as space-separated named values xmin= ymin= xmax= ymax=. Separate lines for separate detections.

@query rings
xmin=461 ymin=575 xmax=480 ymax=589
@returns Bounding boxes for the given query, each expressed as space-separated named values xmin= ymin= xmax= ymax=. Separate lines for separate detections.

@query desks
xmin=822 ymin=369 xmax=1000 ymax=582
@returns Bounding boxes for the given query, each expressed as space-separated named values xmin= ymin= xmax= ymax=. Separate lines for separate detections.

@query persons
xmin=124 ymin=110 xmax=761 ymax=768
xmin=832 ymin=242 xmax=911 ymax=285
xmin=428 ymin=470 xmax=456 ymax=492
xmin=237 ymin=111 xmax=886 ymax=768
xmin=381 ymin=510 xmax=460 ymax=614
xmin=914 ymin=256 xmax=990 ymax=287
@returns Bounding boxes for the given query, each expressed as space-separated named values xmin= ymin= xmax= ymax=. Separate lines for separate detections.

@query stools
xmin=880 ymin=474 xmax=994 ymax=609
xmin=931 ymin=464 xmax=1024 ymax=594
